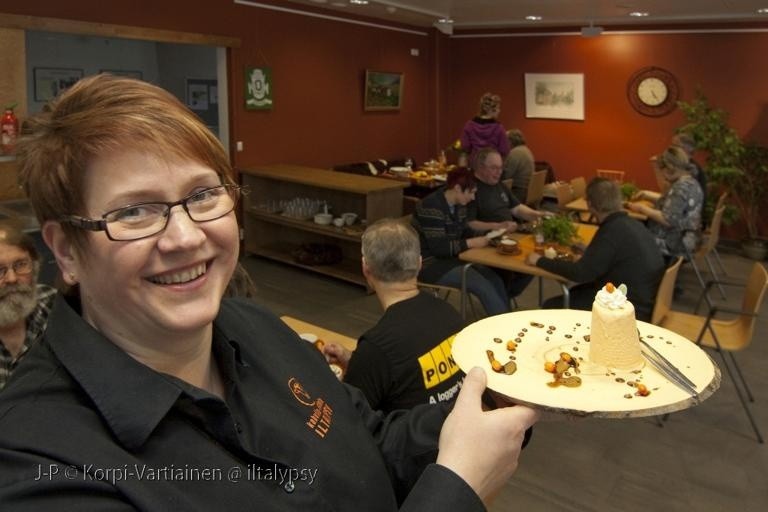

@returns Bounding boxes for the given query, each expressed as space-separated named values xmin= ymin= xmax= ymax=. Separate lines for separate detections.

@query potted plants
xmin=674 ymin=86 xmax=768 ymax=260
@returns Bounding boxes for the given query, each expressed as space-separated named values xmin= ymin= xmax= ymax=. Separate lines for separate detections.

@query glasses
xmin=481 ymin=165 xmax=505 ymax=170
xmin=60 ymin=178 xmax=241 ymax=242
xmin=0 ymin=260 xmax=38 ymax=277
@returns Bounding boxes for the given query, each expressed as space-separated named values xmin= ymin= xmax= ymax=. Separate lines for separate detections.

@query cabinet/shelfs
xmin=238 ymin=162 xmax=412 ymax=295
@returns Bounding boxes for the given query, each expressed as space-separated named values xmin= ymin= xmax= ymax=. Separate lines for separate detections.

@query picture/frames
xmin=33 ymin=66 xmax=83 ymax=103
xmin=525 ymin=72 xmax=585 ymax=121
xmin=365 ymin=68 xmax=406 ymax=110
xmin=245 ymin=68 xmax=272 ymax=110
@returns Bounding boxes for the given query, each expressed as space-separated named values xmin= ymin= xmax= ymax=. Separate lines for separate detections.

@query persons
xmin=454 ymin=91 xmax=512 ymax=155
xmin=1 ymin=224 xmax=58 ymax=390
xmin=321 ymin=216 xmax=468 ymax=416
xmin=400 ymin=154 xmax=418 ymax=172
xmin=503 ymin=128 xmax=536 ymax=203
xmin=629 ymin=145 xmax=703 ymax=293
xmin=672 ymin=134 xmax=707 ymax=213
xmin=466 ymin=147 xmax=556 ymax=299
xmin=409 ymin=168 xmax=510 ymax=316
xmin=0 ymin=84 xmax=541 ymax=512
xmin=526 ymin=176 xmax=666 ymax=322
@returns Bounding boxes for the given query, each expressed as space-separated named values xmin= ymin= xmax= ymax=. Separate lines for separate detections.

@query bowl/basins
xmin=342 ymin=212 xmax=357 ymax=225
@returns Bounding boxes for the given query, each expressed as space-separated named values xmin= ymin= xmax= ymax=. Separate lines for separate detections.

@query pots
xmin=314 ymin=203 xmax=333 ymax=225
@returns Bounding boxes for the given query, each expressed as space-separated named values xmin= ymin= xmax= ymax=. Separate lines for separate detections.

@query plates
xmin=449 ymin=309 xmax=721 ymax=419
xmin=390 ymin=166 xmax=409 ymax=172
xmin=496 ymin=247 xmax=522 ymax=256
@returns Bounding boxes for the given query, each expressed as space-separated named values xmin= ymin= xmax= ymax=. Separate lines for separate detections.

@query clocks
xmin=627 ymin=68 xmax=679 ymax=116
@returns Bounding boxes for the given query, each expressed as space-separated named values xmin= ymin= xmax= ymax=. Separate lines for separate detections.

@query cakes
xmin=588 ymin=282 xmax=644 ymax=372
xmin=544 ymin=242 xmax=559 ymax=259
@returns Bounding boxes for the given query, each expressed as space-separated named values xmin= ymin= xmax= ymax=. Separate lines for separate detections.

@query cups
xmin=300 ymin=333 xmax=325 ymax=351
xmin=497 ymin=239 xmax=518 ymax=252
xmin=542 ymin=241 xmax=559 ymax=261
xmin=333 ymin=218 xmax=344 ymax=227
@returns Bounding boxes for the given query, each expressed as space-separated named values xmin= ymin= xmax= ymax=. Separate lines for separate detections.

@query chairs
xmin=658 ymin=261 xmax=768 ymax=443
xmin=650 ymin=256 xmax=685 ymax=325
xmin=684 ymin=206 xmax=725 ymax=313
xmin=555 ymin=186 xmax=574 ymax=217
xmin=397 ymin=213 xmax=477 ymax=321
xmin=524 ymin=171 xmax=547 ymax=207
xmin=705 ymin=191 xmax=730 ymax=279
xmin=569 ymin=177 xmax=588 ymax=199
